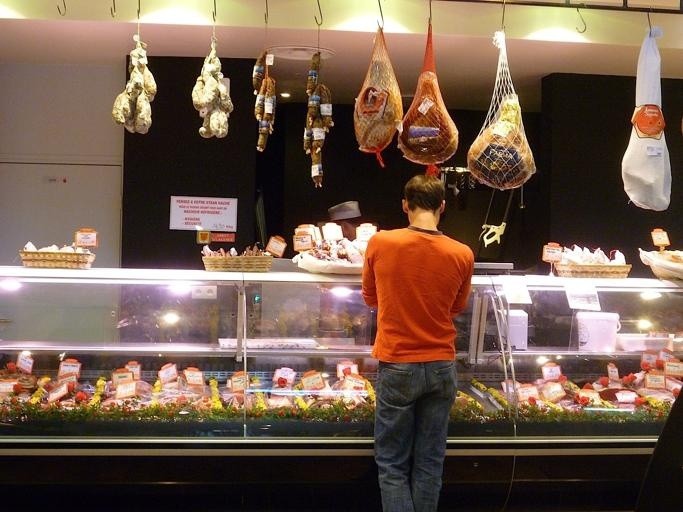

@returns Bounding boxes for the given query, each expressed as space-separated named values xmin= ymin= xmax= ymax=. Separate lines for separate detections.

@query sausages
xmin=304 ymin=54 xmax=334 ymax=188
xmin=252 ymin=52 xmax=276 ymax=153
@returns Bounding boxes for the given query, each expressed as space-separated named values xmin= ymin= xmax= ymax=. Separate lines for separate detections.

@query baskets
xmin=201 ymin=254 xmax=274 ymax=272
xmin=17 ymin=248 xmax=96 ymax=270
xmin=646 ymin=259 xmax=683 ymax=281
xmin=553 ymin=262 xmax=632 ymax=278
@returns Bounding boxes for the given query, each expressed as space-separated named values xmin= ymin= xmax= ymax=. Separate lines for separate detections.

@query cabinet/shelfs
xmin=0 ymin=265 xmax=682 ymax=511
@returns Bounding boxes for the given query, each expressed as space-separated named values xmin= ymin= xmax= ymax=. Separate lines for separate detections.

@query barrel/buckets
xmin=577 ymin=311 xmax=621 ymax=353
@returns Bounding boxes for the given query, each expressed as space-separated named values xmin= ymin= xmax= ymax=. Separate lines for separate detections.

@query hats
xmin=326 ymin=200 xmax=362 ymax=221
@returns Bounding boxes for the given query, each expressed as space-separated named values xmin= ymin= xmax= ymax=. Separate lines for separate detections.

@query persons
xmin=328 ymin=199 xmax=361 ymax=242
xmin=361 ymin=174 xmax=475 ymax=511
